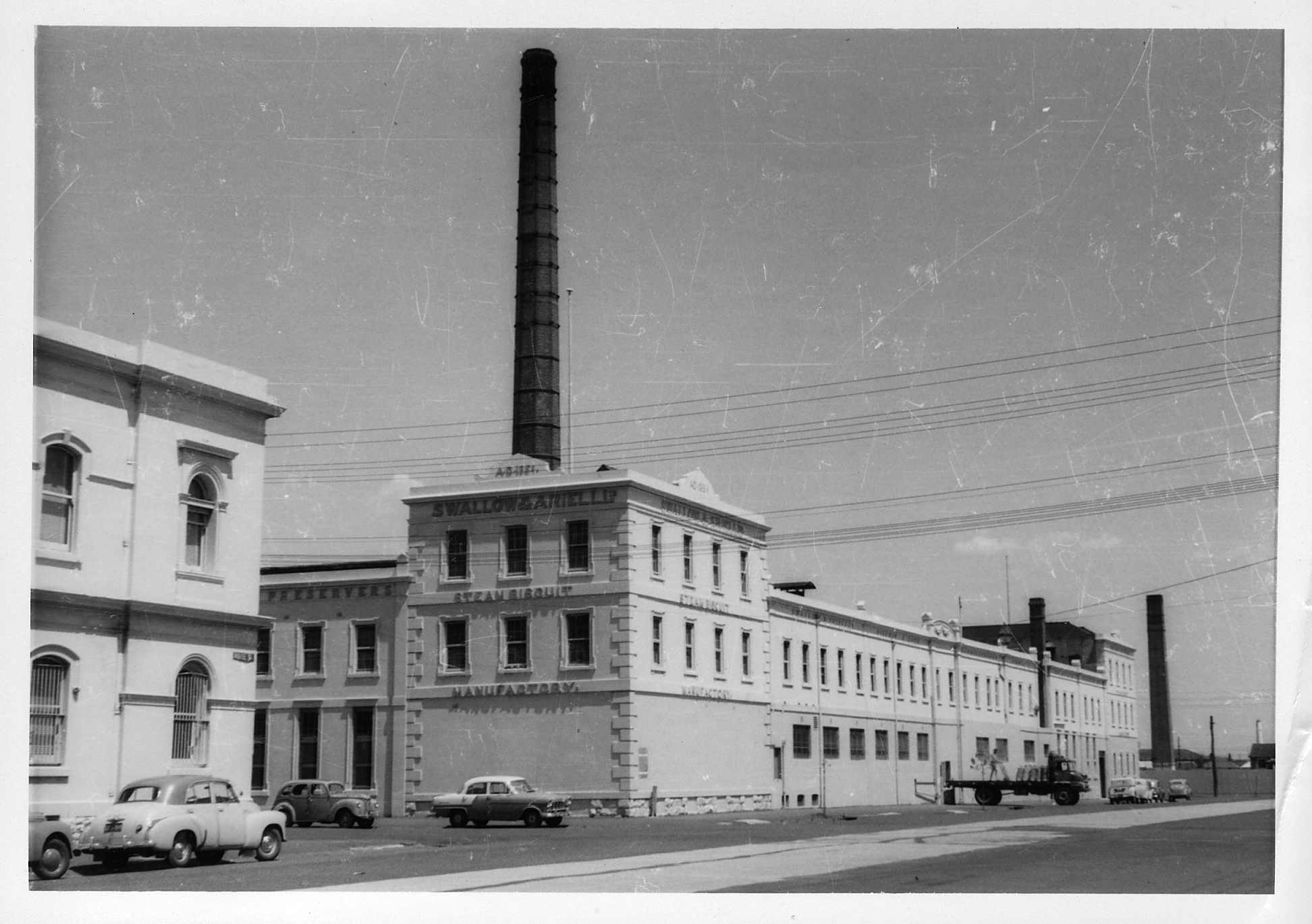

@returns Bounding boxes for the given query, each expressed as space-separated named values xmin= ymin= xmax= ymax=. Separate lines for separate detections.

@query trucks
xmin=942 ymin=751 xmax=1090 ymax=806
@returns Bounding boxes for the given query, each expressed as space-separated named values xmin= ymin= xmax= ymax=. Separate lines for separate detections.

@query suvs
xmin=1107 ymin=778 xmax=1140 ymax=805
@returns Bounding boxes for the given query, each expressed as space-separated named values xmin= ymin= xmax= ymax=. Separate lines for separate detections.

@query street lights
xmin=1255 ymin=719 xmax=1263 ymax=743
xmin=1005 ymin=554 xmax=1009 ymax=625
xmin=566 ymin=288 xmax=573 ymax=471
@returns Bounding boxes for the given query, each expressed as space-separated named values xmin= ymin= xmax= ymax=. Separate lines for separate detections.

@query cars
xmin=1134 ymin=778 xmax=1155 ymax=804
xmin=28 ymin=812 xmax=74 ymax=880
xmin=1149 ymin=779 xmax=1166 ymax=803
xmin=74 ymin=775 xmax=287 ymax=869
xmin=271 ymin=780 xmax=380 ymax=829
xmin=1167 ymin=779 xmax=1194 ymax=801
xmin=426 ymin=776 xmax=572 ymax=828
